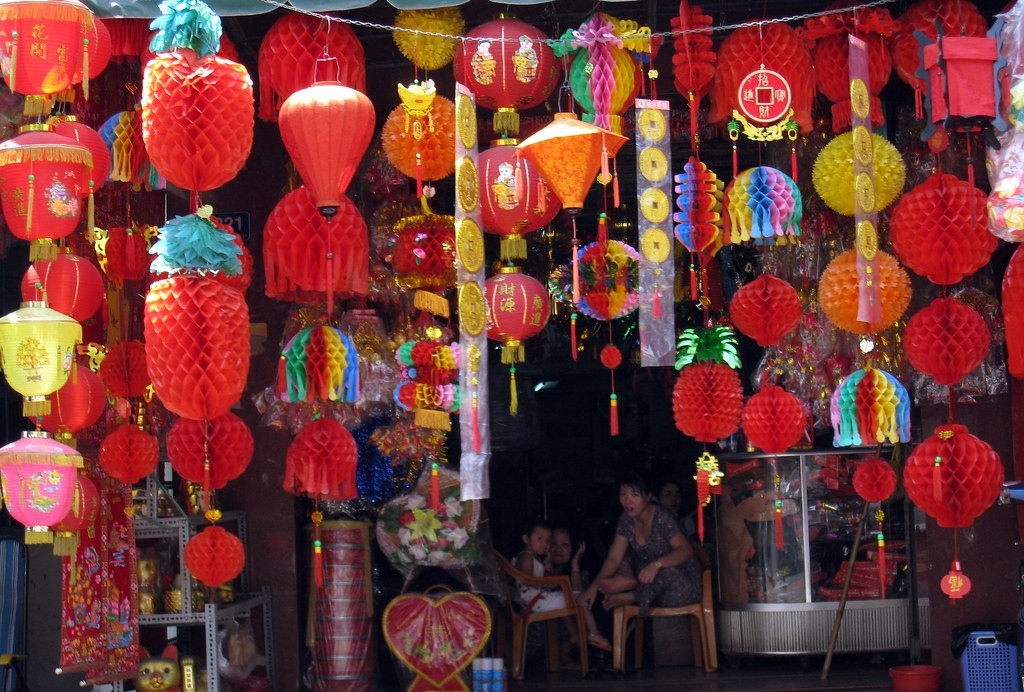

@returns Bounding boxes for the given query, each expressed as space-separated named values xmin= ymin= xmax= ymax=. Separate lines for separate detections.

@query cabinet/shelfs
xmin=113 ymin=453 xmax=275 ymax=692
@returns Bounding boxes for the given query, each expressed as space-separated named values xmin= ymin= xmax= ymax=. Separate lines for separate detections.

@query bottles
xmin=492 ymin=658 xmax=504 ymax=692
xmin=472 ymin=658 xmax=481 ymax=692
xmin=481 ymin=658 xmax=493 ymax=692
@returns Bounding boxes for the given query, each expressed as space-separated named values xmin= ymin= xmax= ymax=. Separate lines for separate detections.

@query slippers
xmin=588 ymin=638 xmax=612 ymax=651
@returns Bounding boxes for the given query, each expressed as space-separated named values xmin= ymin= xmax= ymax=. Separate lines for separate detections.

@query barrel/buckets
xmin=890 ymin=665 xmax=944 ymax=692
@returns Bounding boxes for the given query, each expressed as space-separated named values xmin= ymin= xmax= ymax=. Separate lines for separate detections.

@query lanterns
xmin=0 ymin=0 xmax=1024 ymax=690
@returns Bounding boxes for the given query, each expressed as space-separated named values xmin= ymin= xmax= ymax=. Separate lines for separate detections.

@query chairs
xmin=479 ymin=542 xmax=591 ymax=681
xmin=0 ymin=538 xmax=30 ymax=692
xmin=614 ymin=540 xmax=718 ymax=673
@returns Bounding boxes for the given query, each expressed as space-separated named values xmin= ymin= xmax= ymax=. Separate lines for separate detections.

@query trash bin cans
xmin=960 ymin=623 xmax=1020 ymax=692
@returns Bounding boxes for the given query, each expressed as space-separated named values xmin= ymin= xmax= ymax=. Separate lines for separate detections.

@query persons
xmin=550 ymin=528 xmax=590 ymax=670
xmin=513 ymin=523 xmax=613 ymax=670
xmin=643 ymin=472 xmax=713 ymax=568
xmin=578 ymin=475 xmax=703 ymax=610
